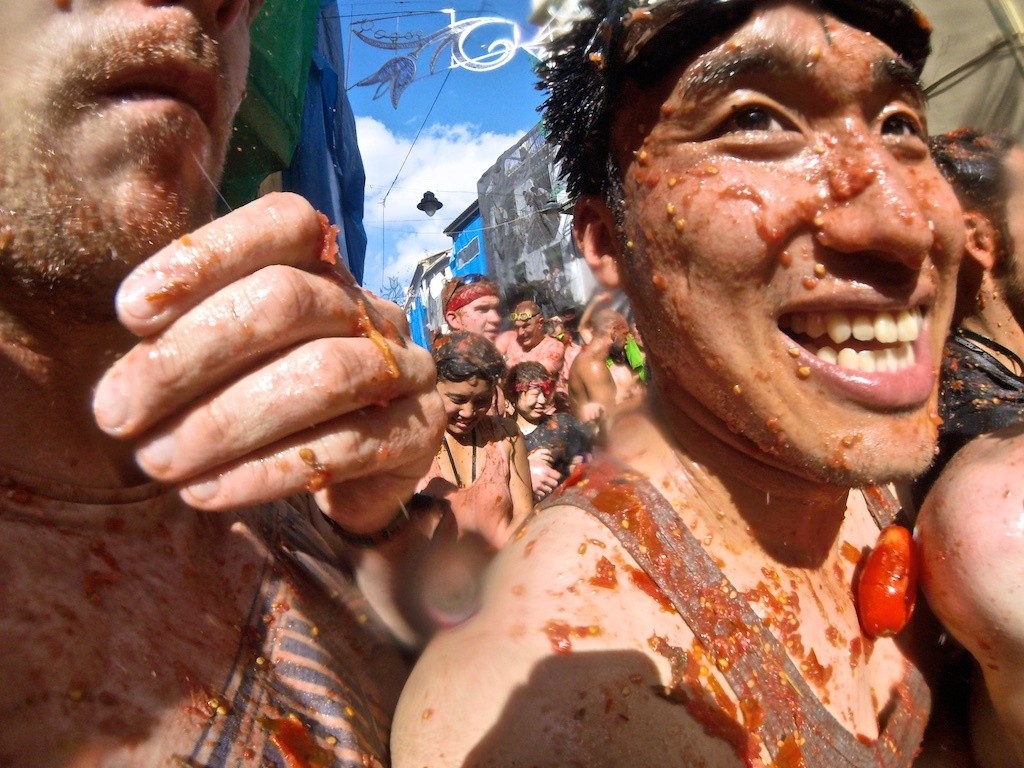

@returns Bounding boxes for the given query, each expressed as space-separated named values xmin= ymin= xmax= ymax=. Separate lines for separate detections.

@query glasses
xmin=446 ymin=273 xmax=487 ymax=306
xmin=434 ymin=356 xmax=503 ymax=382
xmin=553 ymin=333 xmax=567 ymax=342
xmin=510 ymin=311 xmax=541 ymax=321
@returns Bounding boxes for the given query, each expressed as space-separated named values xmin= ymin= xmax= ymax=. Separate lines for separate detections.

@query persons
xmin=415 ymin=330 xmax=535 ymax=547
xmin=0 ymin=0 xmax=1024 ymax=768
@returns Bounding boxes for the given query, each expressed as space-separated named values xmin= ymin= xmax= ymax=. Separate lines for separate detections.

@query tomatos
xmin=857 ymin=524 xmax=919 ymax=635
